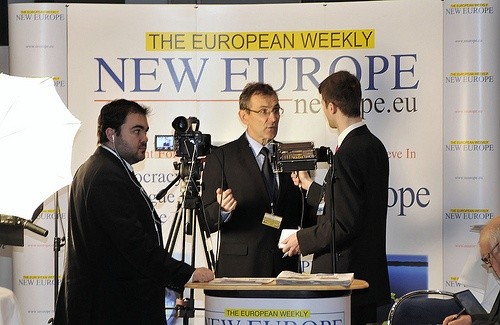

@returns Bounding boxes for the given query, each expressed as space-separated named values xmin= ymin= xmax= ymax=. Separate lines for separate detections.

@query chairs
xmin=388 ymin=289 xmax=463 ymax=325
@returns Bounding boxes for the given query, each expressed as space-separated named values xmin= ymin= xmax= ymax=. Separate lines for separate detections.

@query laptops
xmin=454 ymin=289 xmax=488 ymax=315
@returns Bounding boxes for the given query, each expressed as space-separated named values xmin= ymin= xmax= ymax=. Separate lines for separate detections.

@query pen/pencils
xmin=282 ymin=252 xmax=288 ymax=258
xmin=451 ymin=308 xmax=465 ymax=321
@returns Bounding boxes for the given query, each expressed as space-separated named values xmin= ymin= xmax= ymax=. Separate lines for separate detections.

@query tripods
xmin=164 ymin=182 xmax=217 ymax=325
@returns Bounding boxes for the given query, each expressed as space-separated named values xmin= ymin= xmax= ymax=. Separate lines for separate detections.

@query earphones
xmin=112 ymin=135 xmax=115 ymax=142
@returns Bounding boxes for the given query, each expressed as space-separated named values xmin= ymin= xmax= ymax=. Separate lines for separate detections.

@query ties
xmin=260 ymin=147 xmax=278 ymax=205
xmin=336 ymin=145 xmax=340 ymax=152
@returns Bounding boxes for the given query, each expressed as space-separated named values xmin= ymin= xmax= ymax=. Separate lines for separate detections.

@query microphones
xmin=294 ymin=170 xmax=302 ymax=188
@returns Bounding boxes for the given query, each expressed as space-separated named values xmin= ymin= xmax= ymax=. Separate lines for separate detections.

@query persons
xmin=282 ymin=70 xmax=391 ymax=325
xmin=443 ymin=217 xmax=500 ymax=325
xmin=52 ymin=98 xmax=214 ymax=325
xmin=162 ymin=140 xmax=170 ymax=150
xmin=199 ymin=82 xmax=318 ymax=278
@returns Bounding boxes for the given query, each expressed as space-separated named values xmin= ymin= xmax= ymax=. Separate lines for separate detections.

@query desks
xmin=184 ymin=278 xmax=369 ymax=325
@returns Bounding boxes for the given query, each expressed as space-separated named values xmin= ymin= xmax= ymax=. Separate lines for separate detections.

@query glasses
xmin=243 ymin=107 xmax=284 ymax=115
xmin=481 ymin=242 xmax=500 ymax=264
